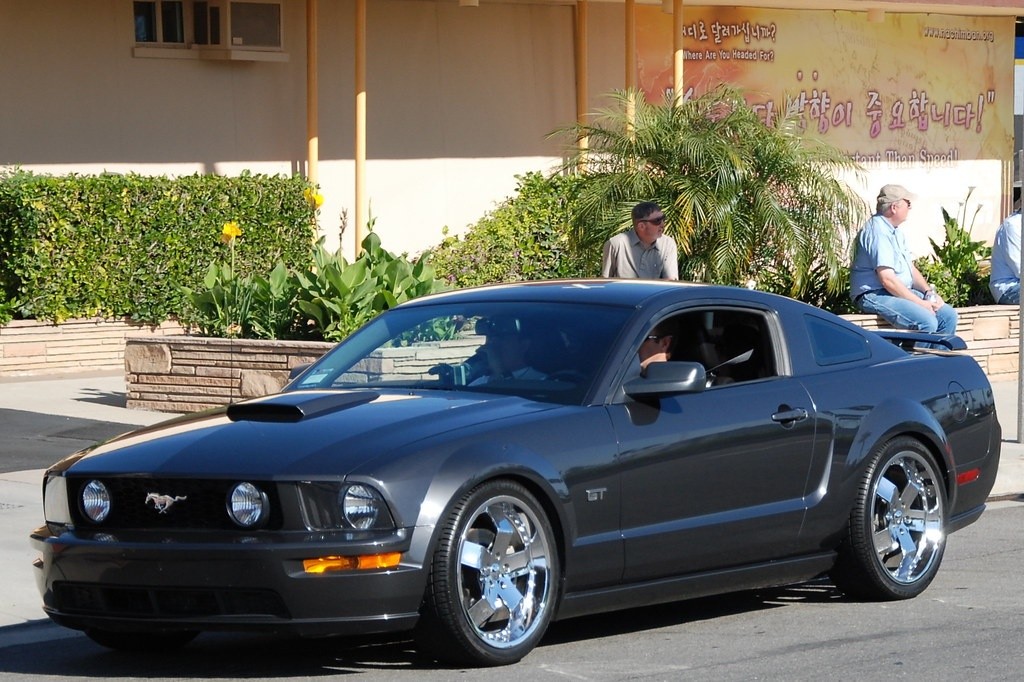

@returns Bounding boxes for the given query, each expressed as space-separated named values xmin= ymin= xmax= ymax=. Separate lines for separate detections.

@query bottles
xmin=924 ymin=284 xmax=937 ymax=310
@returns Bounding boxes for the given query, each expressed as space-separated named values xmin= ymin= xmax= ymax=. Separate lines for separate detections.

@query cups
xmin=453 ymin=366 xmax=466 ymax=385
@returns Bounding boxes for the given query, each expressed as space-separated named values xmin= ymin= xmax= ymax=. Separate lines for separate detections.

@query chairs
xmin=528 ymin=331 xmax=581 ymax=406
xmin=670 ymin=314 xmax=734 ymax=386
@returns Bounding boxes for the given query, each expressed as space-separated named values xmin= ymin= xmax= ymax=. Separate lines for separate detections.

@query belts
xmin=854 ymin=290 xmax=873 ymax=304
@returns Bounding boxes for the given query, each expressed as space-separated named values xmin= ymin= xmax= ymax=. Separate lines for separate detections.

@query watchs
xmin=924 ymin=289 xmax=934 ymax=295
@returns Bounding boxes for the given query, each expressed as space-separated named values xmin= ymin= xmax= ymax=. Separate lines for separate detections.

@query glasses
xmin=637 ymin=214 xmax=666 ymax=225
xmin=643 ymin=334 xmax=658 ymax=344
xmin=903 ymin=198 xmax=911 ymax=206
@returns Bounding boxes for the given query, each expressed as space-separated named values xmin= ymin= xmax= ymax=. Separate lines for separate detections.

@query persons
xmin=989 ymin=195 xmax=1021 ymax=305
xmin=602 ymin=203 xmax=678 ymax=280
xmin=638 ymin=316 xmax=735 ymax=388
xmin=465 ymin=316 xmax=547 ymax=386
xmin=850 ymin=184 xmax=957 ymax=350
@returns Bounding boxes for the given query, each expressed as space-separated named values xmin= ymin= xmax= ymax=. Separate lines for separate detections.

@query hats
xmin=878 ymin=184 xmax=914 ymax=204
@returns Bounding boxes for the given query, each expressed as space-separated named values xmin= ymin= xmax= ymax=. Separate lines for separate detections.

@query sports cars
xmin=25 ymin=266 xmax=1005 ymax=666
